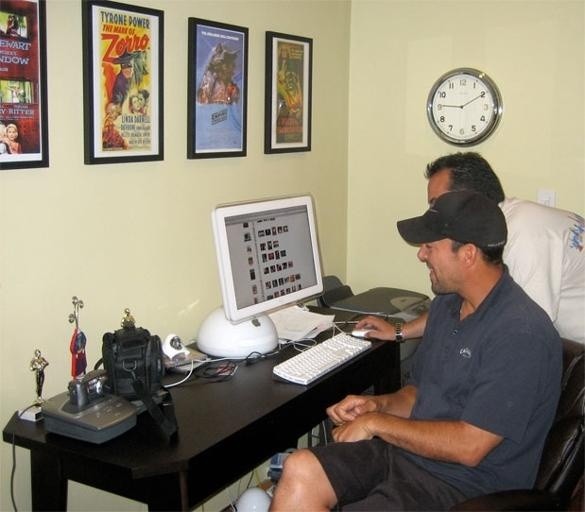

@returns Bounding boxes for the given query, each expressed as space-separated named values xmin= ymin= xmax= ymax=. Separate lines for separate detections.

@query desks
xmin=3 ymin=305 xmax=404 ymax=512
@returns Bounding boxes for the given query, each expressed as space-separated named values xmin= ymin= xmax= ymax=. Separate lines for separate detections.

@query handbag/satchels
xmin=102 ymin=327 xmax=163 ymax=402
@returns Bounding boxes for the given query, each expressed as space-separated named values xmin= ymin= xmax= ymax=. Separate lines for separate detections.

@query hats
xmin=396 ymin=189 xmax=508 ymax=250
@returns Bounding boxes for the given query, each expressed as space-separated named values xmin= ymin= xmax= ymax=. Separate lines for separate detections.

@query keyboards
xmin=272 ymin=331 xmax=372 ymax=385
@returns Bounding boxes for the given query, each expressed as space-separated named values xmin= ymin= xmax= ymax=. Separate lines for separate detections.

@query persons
xmin=0 ymin=123 xmax=22 ymax=156
xmin=6 ymin=15 xmax=19 ymax=37
xmin=101 ymin=102 xmax=127 ymax=151
xmin=110 ymin=47 xmax=147 ymax=118
xmin=266 ymin=190 xmax=563 ymax=512
xmin=355 ymin=153 xmax=585 ymax=348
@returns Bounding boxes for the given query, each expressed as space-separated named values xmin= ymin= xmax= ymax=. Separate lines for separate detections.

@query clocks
xmin=426 ymin=68 xmax=502 ymax=148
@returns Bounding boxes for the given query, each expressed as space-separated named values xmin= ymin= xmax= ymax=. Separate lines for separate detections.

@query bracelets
xmin=394 ymin=322 xmax=404 ymax=343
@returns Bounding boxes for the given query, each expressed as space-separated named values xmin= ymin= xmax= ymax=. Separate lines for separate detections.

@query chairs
xmin=448 ymin=337 xmax=584 ymax=512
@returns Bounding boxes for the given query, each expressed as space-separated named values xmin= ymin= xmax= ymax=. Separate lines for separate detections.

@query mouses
xmin=352 ymin=326 xmax=376 ymax=338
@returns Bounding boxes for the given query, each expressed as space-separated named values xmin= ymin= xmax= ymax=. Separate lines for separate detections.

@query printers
xmin=318 ymin=276 xmax=432 ymax=323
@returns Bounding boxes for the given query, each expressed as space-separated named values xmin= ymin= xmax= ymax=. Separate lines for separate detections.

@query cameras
xmin=68 ymin=369 xmax=109 ymax=406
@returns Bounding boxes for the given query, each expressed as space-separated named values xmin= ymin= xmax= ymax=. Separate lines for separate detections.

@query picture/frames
xmin=187 ymin=17 xmax=248 ymax=158
xmin=265 ymin=30 xmax=312 ymax=154
xmin=0 ymin=0 xmax=49 ymax=171
xmin=82 ymin=0 xmax=164 ymax=165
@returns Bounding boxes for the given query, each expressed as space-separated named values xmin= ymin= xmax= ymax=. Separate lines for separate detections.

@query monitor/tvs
xmin=196 ymin=195 xmax=326 ymax=360
